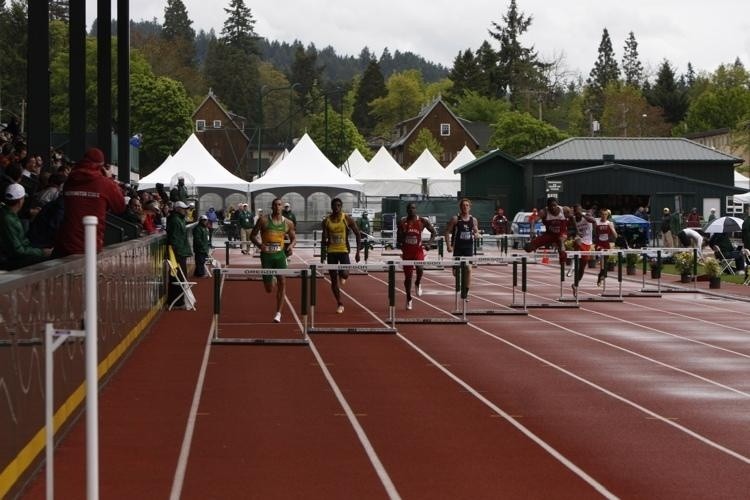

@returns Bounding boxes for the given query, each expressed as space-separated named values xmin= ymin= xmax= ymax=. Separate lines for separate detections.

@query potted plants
xmin=674 ymin=251 xmax=694 ymax=283
xmin=587 ymin=253 xmax=664 ymax=278
xmin=702 ymin=256 xmax=724 ymax=290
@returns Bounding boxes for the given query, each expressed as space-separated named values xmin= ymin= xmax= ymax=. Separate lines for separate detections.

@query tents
xmin=136 ymin=132 xmax=250 ymax=214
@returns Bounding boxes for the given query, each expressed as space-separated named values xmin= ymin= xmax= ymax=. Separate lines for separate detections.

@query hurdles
xmin=559 ymin=250 xmax=624 ymax=301
xmin=313 ymin=230 xmax=324 ymax=257
xmin=451 ymin=256 xmax=529 ymax=315
xmin=509 ymin=253 xmax=582 ymax=308
xmin=212 ymin=268 xmax=311 ymax=346
xmin=349 ymin=234 xmax=538 ymax=275
xmin=384 ymin=259 xmax=470 ymax=325
xmin=223 ymin=241 xmax=264 ymax=281
xmin=307 ymin=264 xmax=398 ymax=334
xmin=640 ymin=246 xmax=698 ymax=293
xmin=599 ymin=248 xmax=662 ymax=298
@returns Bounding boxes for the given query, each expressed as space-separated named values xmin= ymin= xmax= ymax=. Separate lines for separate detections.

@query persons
xmin=395 ymin=203 xmax=439 ymax=312
xmin=206 ymin=208 xmax=217 ymax=247
xmin=106 ymin=164 xmax=171 ymax=237
xmin=247 ymin=198 xmax=297 ymax=322
xmin=318 ymin=198 xmax=361 ymax=315
xmin=165 ymin=202 xmax=192 ymax=309
xmin=193 ymin=214 xmax=209 ymax=277
xmin=217 ymin=203 xmax=261 ymax=256
xmin=58 ymin=147 xmax=126 ymax=256
xmin=355 ymin=211 xmax=373 ymax=264
xmin=184 ymin=203 xmax=198 ymax=224
xmin=487 ymin=196 xmax=750 ymax=302
xmin=278 ymin=202 xmax=297 ymax=229
xmin=1 ymin=132 xmax=71 ymax=269
xmin=443 ymin=199 xmax=484 ymax=303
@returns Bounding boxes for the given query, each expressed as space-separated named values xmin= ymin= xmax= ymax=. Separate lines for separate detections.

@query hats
xmin=84 ymin=147 xmax=105 ymax=167
xmin=5 ymin=183 xmax=29 ymax=200
xmin=173 ymin=201 xmax=190 ymax=209
xmin=198 ymin=215 xmax=208 ymax=220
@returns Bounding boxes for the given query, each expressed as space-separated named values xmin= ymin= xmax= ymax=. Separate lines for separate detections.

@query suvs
xmin=509 ymin=210 xmax=546 ymax=249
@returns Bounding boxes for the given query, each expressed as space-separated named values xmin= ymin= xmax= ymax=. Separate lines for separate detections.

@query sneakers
xmin=572 ymin=284 xmax=576 ymax=297
xmin=415 ymin=283 xmax=423 ymax=296
xmin=274 ymin=312 xmax=281 ymax=323
xmin=567 ymin=269 xmax=573 ymax=277
xmin=597 ymin=275 xmax=603 ymax=287
xmin=406 ymin=299 xmax=412 ymax=310
xmin=340 ymin=276 xmax=345 ymax=286
xmin=284 ymin=202 xmax=290 ymax=207
xmin=336 ymin=305 xmax=344 ymax=313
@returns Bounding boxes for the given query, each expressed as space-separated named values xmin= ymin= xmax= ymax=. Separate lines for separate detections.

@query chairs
xmin=712 ymin=244 xmax=735 ymax=276
xmin=742 ymin=247 xmax=750 ymax=286
xmin=166 ymin=255 xmax=198 ymax=315
